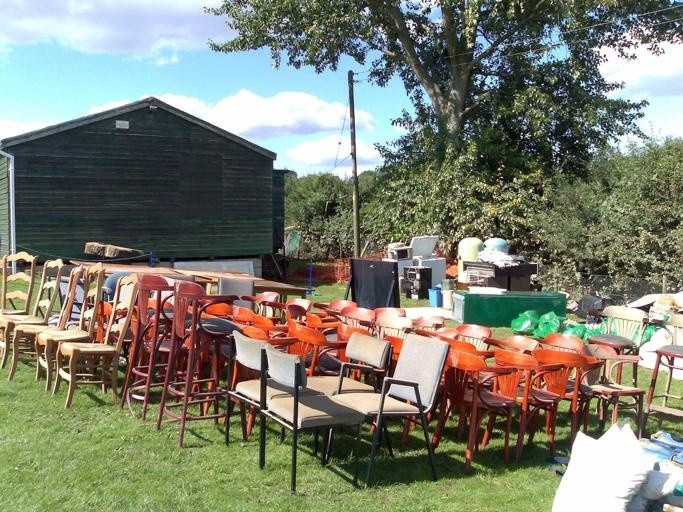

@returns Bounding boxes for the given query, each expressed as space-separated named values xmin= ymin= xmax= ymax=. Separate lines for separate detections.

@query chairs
xmin=200 ymin=305 xmax=254 ymax=427
xmin=327 ymin=299 xmax=358 ymax=322
xmin=7 ymin=263 xmax=85 ymax=384
xmin=282 ymin=308 xmax=364 ymax=382
xmin=92 ymin=301 xmax=113 ymax=347
xmin=285 ymin=304 xmax=305 ymax=321
xmin=568 ymin=343 xmax=646 ymax=444
xmin=0 ymin=258 xmax=65 ymax=371
xmin=217 ymin=277 xmax=254 ymax=308
xmin=51 ymin=272 xmax=140 ymax=410
xmin=304 ymin=330 xmax=394 ymax=443
xmin=336 ymin=322 xmax=368 ymax=381
xmin=304 ymin=311 xmax=338 ymax=336
xmin=185 ymin=300 xmax=234 ymax=418
xmin=339 ymin=307 xmax=376 ymax=332
xmin=430 ymin=348 xmax=517 ymax=473
xmin=542 ymin=334 xmax=587 ymax=382
xmin=368 ymin=334 xmax=434 ymax=431
xmin=373 ymin=307 xmax=407 ymax=318
xmin=163 ymin=273 xmax=195 ymax=283
xmin=433 ymin=326 xmax=458 ymax=342
xmin=323 ymin=332 xmax=450 ymax=490
xmin=34 ymin=262 xmax=107 ymax=392
xmin=120 ymin=272 xmax=205 ymax=422
xmin=454 ymin=322 xmax=489 ymax=350
xmin=375 ymin=316 xmax=414 ymax=368
xmin=156 ymin=279 xmax=249 ymax=448
xmin=0 ymin=251 xmax=38 ymax=358
xmin=414 ymin=315 xmax=444 ymax=333
xmin=585 ymin=305 xmax=651 ymax=430
xmin=260 ymin=297 xmax=312 ymax=327
xmin=531 ymin=349 xmax=606 ymax=456
xmin=259 ymin=342 xmax=367 ymax=496
xmin=482 ymin=346 xmax=566 ymax=464
xmin=643 ymin=313 xmax=683 ymax=433
xmin=481 ymin=334 xmax=540 ymax=357
xmin=222 ymin=323 xmax=310 ymax=436
xmin=252 ymin=315 xmax=274 ymax=329
xmin=145 ymin=297 xmax=173 ymax=309
xmin=226 ymin=330 xmax=325 ymax=457
xmin=240 ymin=290 xmax=282 ymax=322
xmin=431 ymin=332 xmax=494 ymax=393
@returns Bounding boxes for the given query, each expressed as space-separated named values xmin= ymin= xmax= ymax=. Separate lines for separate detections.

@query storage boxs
xmin=404 ymin=266 xmax=433 ymax=282
xmin=405 ymin=281 xmax=432 ymax=299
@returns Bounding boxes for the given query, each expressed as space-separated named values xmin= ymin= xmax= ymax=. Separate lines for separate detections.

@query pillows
xmin=621 ymin=422 xmax=639 ymax=444
xmin=549 ymin=430 xmax=659 ymax=512
xmin=597 ymin=423 xmax=677 ymax=512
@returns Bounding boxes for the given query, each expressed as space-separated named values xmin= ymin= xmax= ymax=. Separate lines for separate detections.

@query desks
xmin=451 ymin=290 xmax=567 ymax=332
xmin=70 ymin=258 xmax=316 ymax=304
